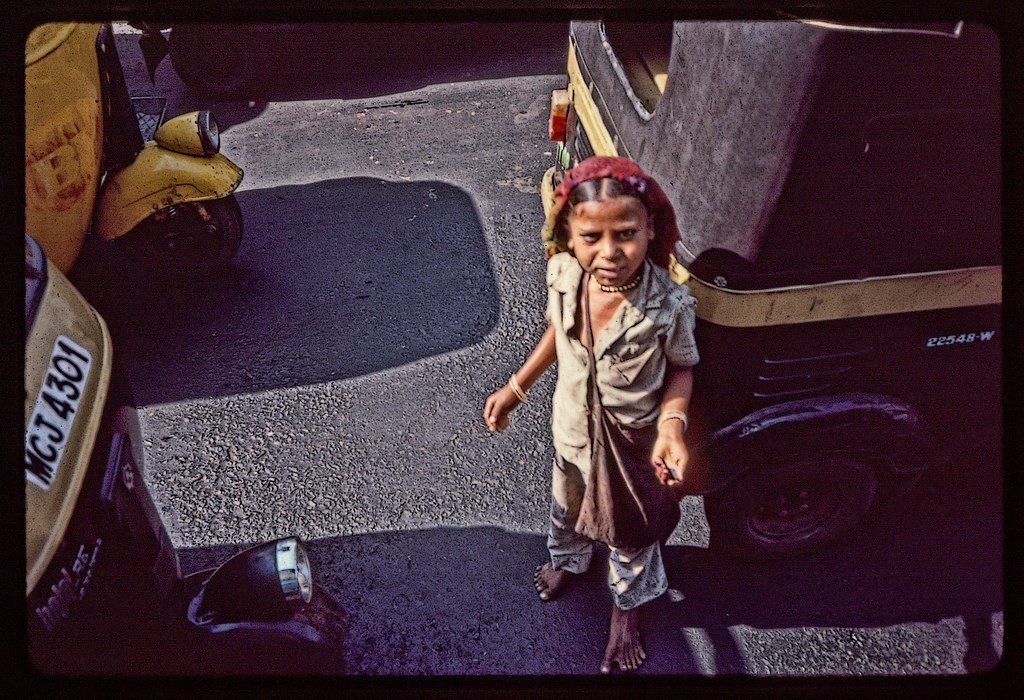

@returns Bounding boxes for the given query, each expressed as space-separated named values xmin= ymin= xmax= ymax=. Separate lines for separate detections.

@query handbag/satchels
xmin=575 ymin=405 xmax=682 ymax=551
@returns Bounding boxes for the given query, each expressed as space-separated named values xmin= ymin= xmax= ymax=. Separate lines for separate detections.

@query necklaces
xmin=591 ymin=274 xmax=641 ymax=292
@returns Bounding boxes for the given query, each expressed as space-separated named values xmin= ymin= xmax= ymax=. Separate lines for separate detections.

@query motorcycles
xmin=537 ymin=14 xmax=1005 ymax=569
xmin=24 ymin=23 xmax=244 ymax=293
xmin=25 ymin=233 xmax=350 ymax=679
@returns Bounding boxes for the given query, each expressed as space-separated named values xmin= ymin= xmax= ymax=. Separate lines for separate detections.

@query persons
xmin=485 ymin=154 xmax=702 ymax=674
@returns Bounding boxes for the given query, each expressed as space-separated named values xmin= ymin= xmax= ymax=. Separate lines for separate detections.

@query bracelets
xmin=657 ymin=410 xmax=687 ymax=435
xmin=508 ymin=374 xmax=525 ymax=402
